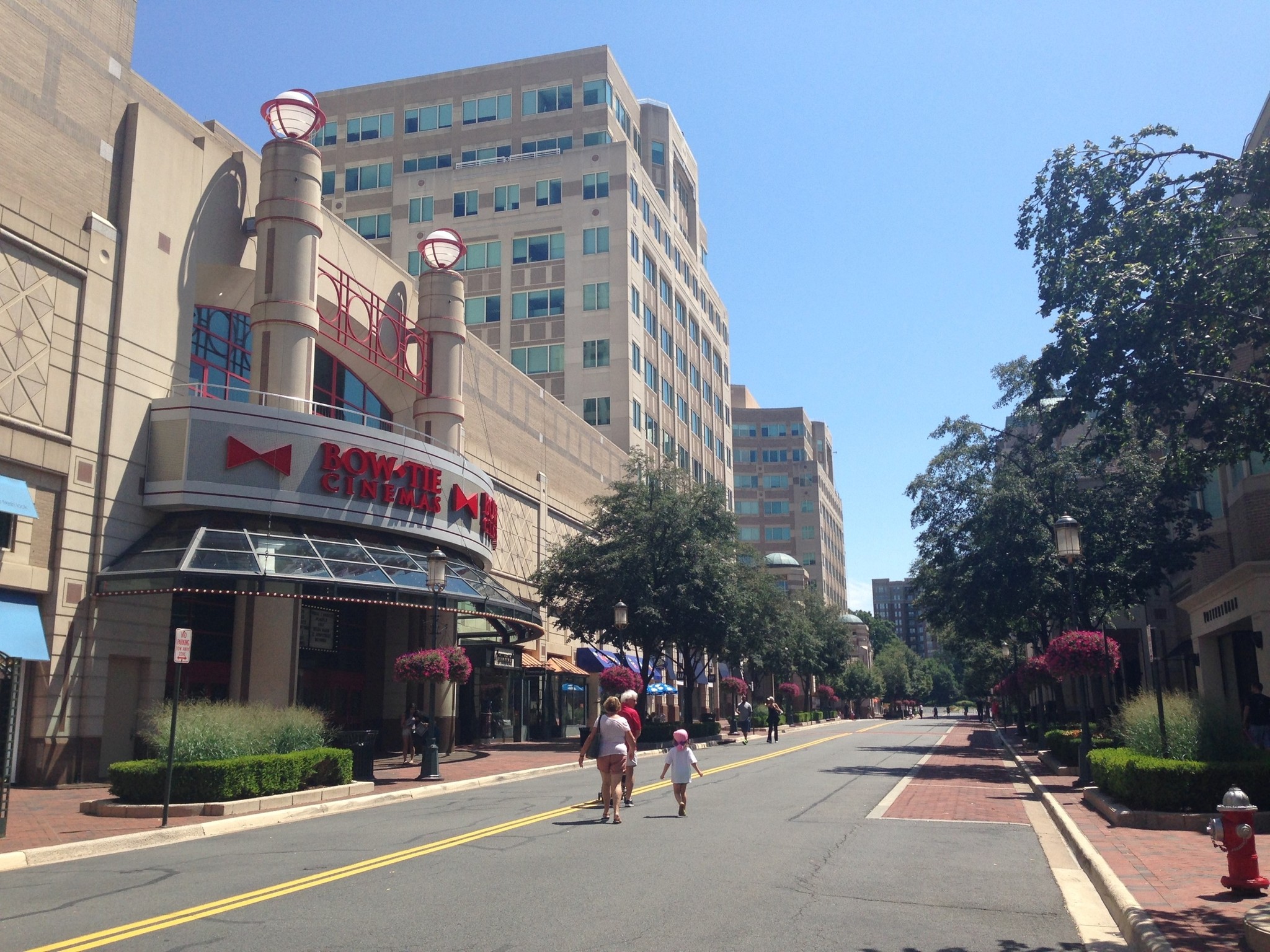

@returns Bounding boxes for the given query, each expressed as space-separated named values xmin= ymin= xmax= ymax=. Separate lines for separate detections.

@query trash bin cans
xmin=334 ymin=729 xmax=379 ymax=781
xmin=701 ymin=712 xmax=716 ymax=724
xmin=578 ymin=727 xmax=592 ymax=746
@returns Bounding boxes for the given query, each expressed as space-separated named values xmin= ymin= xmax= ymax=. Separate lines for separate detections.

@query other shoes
xmin=775 ymin=740 xmax=778 ymax=743
xmin=678 ymin=802 xmax=686 ymax=816
xmin=767 ymin=740 xmax=772 ymax=743
xmin=625 ymin=798 xmax=635 ymax=806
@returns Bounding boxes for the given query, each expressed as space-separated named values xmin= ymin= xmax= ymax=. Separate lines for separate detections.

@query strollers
xmin=598 ymin=773 xmax=626 ymax=805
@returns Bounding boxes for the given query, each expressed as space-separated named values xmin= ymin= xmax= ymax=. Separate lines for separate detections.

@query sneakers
xmin=742 ymin=740 xmax=748 ymax=745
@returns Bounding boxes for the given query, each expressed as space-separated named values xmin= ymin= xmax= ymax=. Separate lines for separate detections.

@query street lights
xmin=612 ymin=599 xmax=628 ymax=667
xmin=1052 ymin=509 xmax=1096 ymax=787
xmin=414 ymin=546 xmax=448 ymax=782
xmin=1009 ymin=629 xmax=1027 ymax=736
xmin=726 ymin=652 xmax=739 ymax=732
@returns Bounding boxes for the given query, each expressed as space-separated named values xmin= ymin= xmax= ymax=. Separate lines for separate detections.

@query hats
xmin=767 ymin=696 xmax=774 ymax=701
xmin=674 ymin=729 xmax=688 ymax=751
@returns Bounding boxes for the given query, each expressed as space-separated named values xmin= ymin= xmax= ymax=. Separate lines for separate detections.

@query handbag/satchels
xmin=774 ymin=704 xmax=784 ymax=716
xmin=586 ymin=714 xmax=603 ymax=760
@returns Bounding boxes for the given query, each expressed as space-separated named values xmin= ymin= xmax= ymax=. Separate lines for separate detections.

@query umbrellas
xmin=562 ymin=683 xmax=583 ymax=692
xmin=647 ymin=683 xmax=678 ymax=715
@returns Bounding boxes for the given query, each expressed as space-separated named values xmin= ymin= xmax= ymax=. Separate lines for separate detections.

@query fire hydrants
xmin=1206 ymin=783 xmax=1270 ymax=895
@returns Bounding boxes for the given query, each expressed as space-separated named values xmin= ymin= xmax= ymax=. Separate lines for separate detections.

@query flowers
xmin=393 ymin=646 xmax=473 ymax=685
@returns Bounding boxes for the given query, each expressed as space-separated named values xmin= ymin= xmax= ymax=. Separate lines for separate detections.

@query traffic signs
xmin=173 ymin=627 xmax=192 ymax=664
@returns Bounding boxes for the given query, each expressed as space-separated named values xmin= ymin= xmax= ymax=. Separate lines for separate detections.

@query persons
xmin=608 ymin=689 xmax=642 ymax=806
xmin=579 ymin=696 xmax=636 ymax=824
xmin=400 ymin=703 xmax=416 ymax=764
xmin=764 ymin=696 xmax=783 ymax=743
xmin=660 ymin=728 xmax=703 ymax=816
xmin=909 ymin=696 xmax=998 ymax=723
xmin=836 ymin=704 xmax=871 ymax=720
xmin=414 ymin=709 xmax=441 ymax=766
xmin=736 ymin=695 xmax=753 ymax=745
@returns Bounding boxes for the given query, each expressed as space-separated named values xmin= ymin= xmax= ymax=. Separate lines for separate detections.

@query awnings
xmin=575 ymin=647 xmax=662 ymax=680
xmin=718 ymin=663 xmax=730 ymax=679
xmin=690 ymin=655 xmax=708 ymax=684
xmin=0 ymin=475 xmax=50 ymax=662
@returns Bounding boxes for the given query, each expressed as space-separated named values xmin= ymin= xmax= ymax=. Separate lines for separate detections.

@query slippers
xmin=613 ymin=818 xmax=622 ymax=824
xmin=601 ymin=813 xmax=610 ymax=820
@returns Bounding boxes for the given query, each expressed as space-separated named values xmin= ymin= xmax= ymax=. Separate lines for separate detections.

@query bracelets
xmin=579 ymin=754 xmax=584 ymax=757
